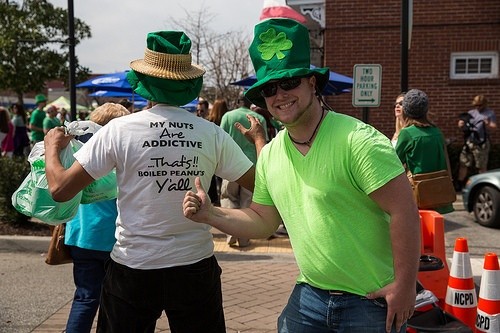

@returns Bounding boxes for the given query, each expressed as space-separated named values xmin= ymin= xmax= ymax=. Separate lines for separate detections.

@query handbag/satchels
xmin=45 ymin=223 xmax=73 ymax=265
xmin=11 ymin=120 xmax=117 ymax=226
xmin=408 ymin=169 xmax=456 ymax=209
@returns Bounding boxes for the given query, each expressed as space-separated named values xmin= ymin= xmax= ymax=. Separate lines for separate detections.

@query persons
xmin=389 ymin=92 xmax=455 ymax=215
xmin=44 ymin=30 xmax=267 ymax=333
xmin=182 ymin=17 xmax=422 ymax=333
xmin=196 ymin=99 xmax=287 ymax=247
xmin=0 ymin=93 xmax=86 ymax=158
xmin=456 ymin=94 xmax=496 ymax=190
xmin=62 ymin=102 xmax=130 ymax=332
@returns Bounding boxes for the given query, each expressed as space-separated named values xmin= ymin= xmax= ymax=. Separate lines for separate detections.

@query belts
xmin=323 ymin=290 xmax=349 ymax=294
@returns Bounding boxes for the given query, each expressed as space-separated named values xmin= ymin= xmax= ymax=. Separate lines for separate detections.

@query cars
xmin=462 ymin=169 xmax=500 ymax=227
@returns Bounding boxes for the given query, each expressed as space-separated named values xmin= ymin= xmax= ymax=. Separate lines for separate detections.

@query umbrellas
xmin=65 ymin=70 xmax=200 ymax=113
xmin=43 ymin=96 xmax=86 ymax=110
xmin=230 ymin=64 xmax=353 ymax=96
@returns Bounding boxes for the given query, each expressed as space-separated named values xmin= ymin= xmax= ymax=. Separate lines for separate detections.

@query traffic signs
xmin=351 ymin=63 xmax=383 ymax=109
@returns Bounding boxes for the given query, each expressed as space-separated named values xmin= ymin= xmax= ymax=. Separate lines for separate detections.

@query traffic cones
xmin=474 ymin=253 xmax=500 ymax=333
xmin=444 ymin=237 xmax=478 ymax=330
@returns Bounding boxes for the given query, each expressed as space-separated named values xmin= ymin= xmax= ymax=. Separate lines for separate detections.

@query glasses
xmin=260 ymin=75 xmax=309 ymax=97
xmin=395 ymin=100 xmax=403 ymax=106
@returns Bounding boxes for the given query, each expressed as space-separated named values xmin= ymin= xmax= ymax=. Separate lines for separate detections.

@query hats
xmin=403 ymin=89 xmax=429 ymax=116
xmin=127 ymin=30 xmax=203 ymax=105
xmin=35 ymin=94 xmax=47 ymax=104
xmin=244 ymin=18 xmax=329 ymax=108
